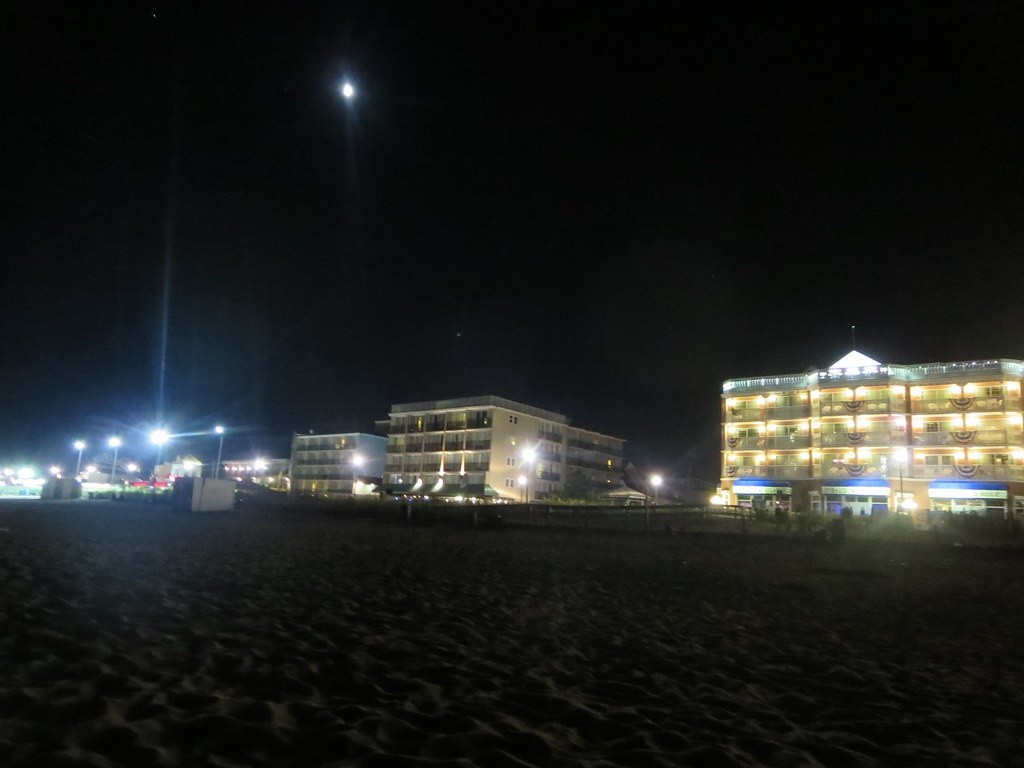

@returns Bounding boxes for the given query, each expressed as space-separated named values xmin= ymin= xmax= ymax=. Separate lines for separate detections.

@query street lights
xmin=513 ymin=439 xmax=544 ymax=503
xmin=75 ymin=442 xmax=84 ymax=476
xmin=109 ymin=438 xmax=120 ymax=484
xmin=215 ymin=425 xmax=224 ymax=479
xmin=649 ymin=473 xmax=664 ymax=503
xmin=150 ymin=430 xmax=170 ymax=465
xmin=354 ymin=457 xmax=362 ymax=483
